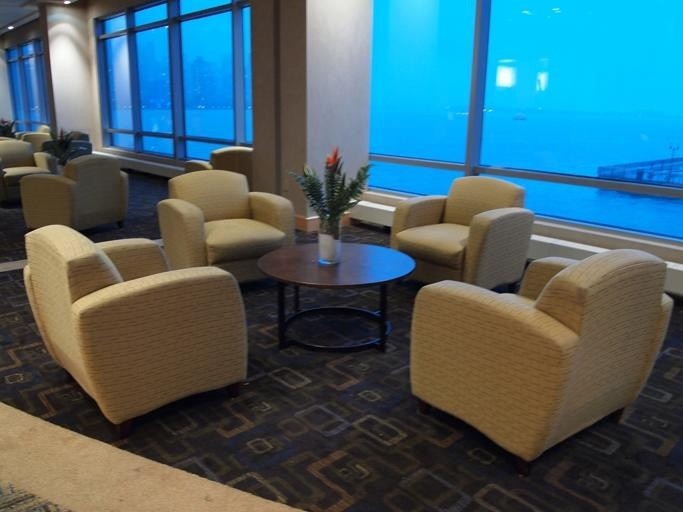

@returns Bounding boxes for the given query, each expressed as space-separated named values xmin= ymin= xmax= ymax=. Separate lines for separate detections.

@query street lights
xmin=668 ymin=145 xmax=681 ymax=184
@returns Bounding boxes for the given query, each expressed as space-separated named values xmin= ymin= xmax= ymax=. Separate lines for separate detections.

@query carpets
xmin=0 ymin=168 xmax=172 ymax=264
xmin=0 ymin=226 xmax=683 ymax=512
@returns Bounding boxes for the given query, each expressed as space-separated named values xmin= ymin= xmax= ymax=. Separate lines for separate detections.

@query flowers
xmin=287 ymin=145 xmax=377 ymax=240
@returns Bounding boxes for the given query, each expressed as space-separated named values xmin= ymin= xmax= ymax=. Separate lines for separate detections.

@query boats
xmin=512 ymin=114 xmax=528 ymax=121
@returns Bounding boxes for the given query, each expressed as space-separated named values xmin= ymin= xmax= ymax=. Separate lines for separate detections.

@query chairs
xmin=156 ymin=170 xmax=297 ymax=284
xmin=409 ymin=248 xmax=674 ymax=463
xmin=22 ymin=224 xmax=249 ymax=438
xmin=185 ymin=146 xmax=255 ymax=192
xmin=390 ymin=176 xmax=535 ymax=291
xmin=0 ymin=124 xmax=129 ymax=234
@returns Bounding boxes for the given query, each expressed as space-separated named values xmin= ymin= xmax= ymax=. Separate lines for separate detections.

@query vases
xmin=318 ymin=217 xmax=341 ymax=266
